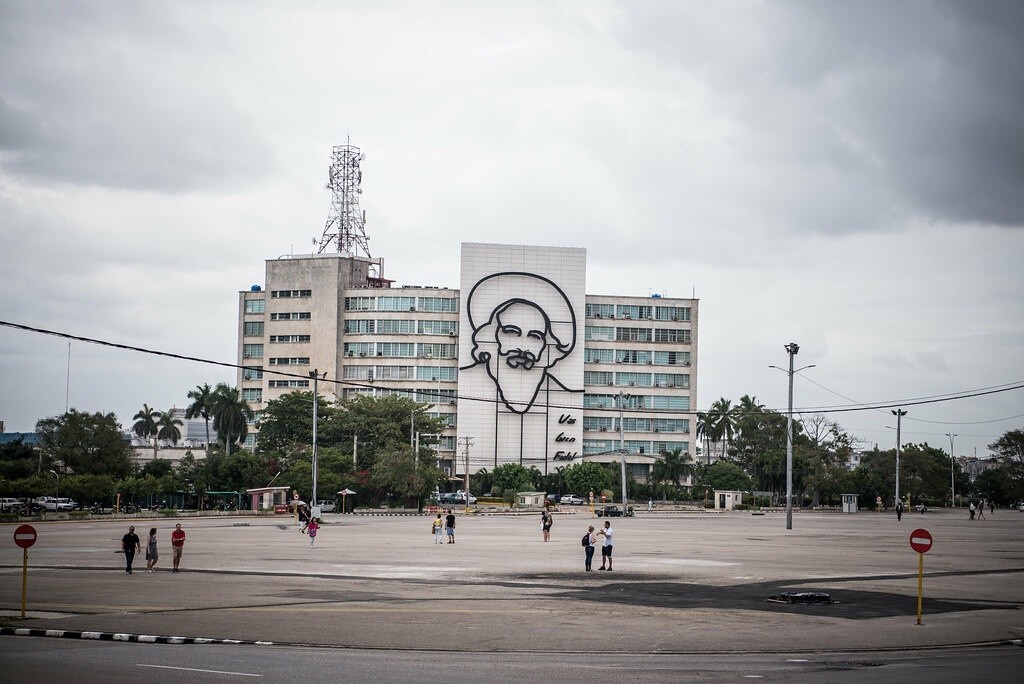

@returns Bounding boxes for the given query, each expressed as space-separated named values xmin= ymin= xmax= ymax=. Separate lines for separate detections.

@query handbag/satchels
xmin=432 ymin=526 xmax=435 ymax=534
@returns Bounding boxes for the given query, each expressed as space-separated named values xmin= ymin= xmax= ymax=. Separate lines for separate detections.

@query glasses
xmin=129 ymin=529 xmax=134 ymax=530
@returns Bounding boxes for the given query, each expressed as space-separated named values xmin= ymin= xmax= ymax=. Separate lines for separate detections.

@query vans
xmin=546 ymin=494 xmax=560 ymax=502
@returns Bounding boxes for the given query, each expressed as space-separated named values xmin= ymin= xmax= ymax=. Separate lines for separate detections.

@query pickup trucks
xmin=594 ymin=506 xmax=623 ymax=517
xmin=38 ymin=498 xmax=73 ymax=512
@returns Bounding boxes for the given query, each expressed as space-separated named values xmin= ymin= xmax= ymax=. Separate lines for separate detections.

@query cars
xmin=58 ymin=498 xmax=78 ymax=508
xmin=36 ymin=497 xmax=54 ymax=502
xmin=560 ymin=494 xmax=583 ymax=505
xmin=316 ymin=500 xmax=336 ymax=513
xmin=1019 ymin=503 xmax=1024 ymax=511
xmin=287 ymin=501 xmax=307 ymax=512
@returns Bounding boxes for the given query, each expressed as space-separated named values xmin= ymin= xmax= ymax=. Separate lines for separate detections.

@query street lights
xmin=885 ymin=425 xmax=900 ymax=507
xmin=769 ymin=365 xmax=817 ymax=529
xmin=946 ymin=433 xmax=958 ymax=507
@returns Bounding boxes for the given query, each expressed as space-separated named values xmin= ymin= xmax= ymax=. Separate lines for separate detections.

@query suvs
xmin=430 ymin=491 xmax=440 ymax=501
xmin=441 ymin=493 xmax=459 ymax=504
xmin=455 ymin=492 xmax=477 ymax=504
xmin=16 ymin=498 xmax=40 ymax=510
xmin=0 ymin=498 xmax=24 ymax=513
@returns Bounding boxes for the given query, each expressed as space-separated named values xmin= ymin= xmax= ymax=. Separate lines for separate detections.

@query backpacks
xmin=582 ymin=533 xmax=592 ymax=546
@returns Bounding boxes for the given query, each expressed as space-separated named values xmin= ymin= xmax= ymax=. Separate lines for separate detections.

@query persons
xmin=969 ymin=501 xmax=986 ymax=520
xmin=990 ymin=503 xmax=995 ymax=514
xmin=585 ymin=521 xmax=614 ymax=571
xmin=433 ymin=510 xmax=456 ymax=544
xmin=896 ymin=503 xmax=903 ymax=521
xmin=648 ymin=499 xmax=653 ymax=512
xmin=121 ymin=525 xmax=141 ymax=574
xmin=920 ymin=503 xmax=925 ymax=514
xmin=171 ymin=524 xmax=185 ymax=573
xmin=298 ymin=506 xmax=320 ymax=548
xmin=145 ymin=528 xmax=159 ymax=573
xmin=541 ymin=508 xmax=553 ymax=542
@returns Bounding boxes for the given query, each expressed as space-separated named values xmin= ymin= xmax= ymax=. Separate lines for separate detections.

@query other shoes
xmin=173 ymin=569 xmax=180 ymax=573
xmin=128 ymin=570 xmax=132 ymax=573
xmin=447 ymin=541 xmax=451 ymax=544
xmin=147 ymin=569 xmax=154 ymax=572
xmin=440 ymin=542 xmax=443 ymax=544
xmin=607 ymin=567 xmax=612 ymax=571
xmin=453 ymin=541 xmax=455 ymax=544
xmin=598 ymin=566 xmax=606 ymax=570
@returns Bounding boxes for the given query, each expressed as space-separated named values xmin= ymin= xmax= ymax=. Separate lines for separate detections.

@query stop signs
xmin=13 ymin=524 xmax=37 ymax=549
xmin=909 ymin=528 xmax=933 ymax=554
xmin=601 ymin=492 xmax=606 ymax=500
